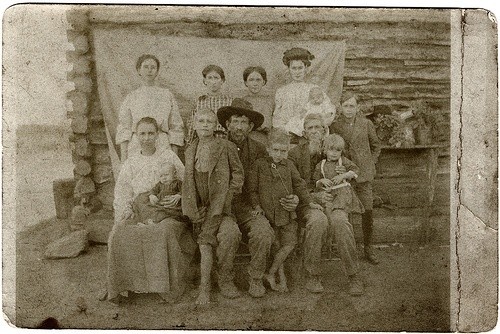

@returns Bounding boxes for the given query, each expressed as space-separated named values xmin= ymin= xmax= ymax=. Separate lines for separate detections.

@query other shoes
xmin=217 ymin=278 xmax=244 ymax=300
xmin=306 ymin=274 xmax=324 ymax=293
xmin=347 ymin=275 xmax=363 ymax=296
xmin=244 ymin=272 xmax=265 ymax=298
xmin=366 ymin=252 xmax=381 ymax=265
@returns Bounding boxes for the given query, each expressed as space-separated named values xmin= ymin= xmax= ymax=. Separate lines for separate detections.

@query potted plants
xmin=410 ymin=98 xmax=446 ymax=145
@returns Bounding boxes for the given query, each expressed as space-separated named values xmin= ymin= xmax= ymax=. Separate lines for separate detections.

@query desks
xmin=374 ymin=142 xmax=444 ymax=250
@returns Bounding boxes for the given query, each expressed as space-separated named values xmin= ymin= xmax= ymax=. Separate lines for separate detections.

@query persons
xmin=330 ymin=91 xmax=381 ymax=265
xmin=114 ymin=55 xmax=186 ymax=161
xmin=286 ymin=113 xmax=365 ymax=296
xmin=187 ymin=64 xmax=235 ymax=144
xmin=313 ymin=132 xmax=365 ymax=263
xmin=284 ymin=86 xmax=331 ymax=138
xmin=193 ymin=99 xmax=300 ymax=298
xmin=241 ymin=66 xmax=274 ymax=131
xmin=125 ymin=161 xmax=185 ymax=227
xmin=181 ymin=105 xmax=244 ymax=307
xmin=246 ymin=128 xmax=324 ymax=294
xmin=104 ymin=118 xmax=194 ymax=306
xmin=271 ymin=48 xmax=338 ymax=145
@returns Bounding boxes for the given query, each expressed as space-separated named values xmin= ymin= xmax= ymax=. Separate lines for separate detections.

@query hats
xmin=216 ymin=97 xmax=264 ymax=131
